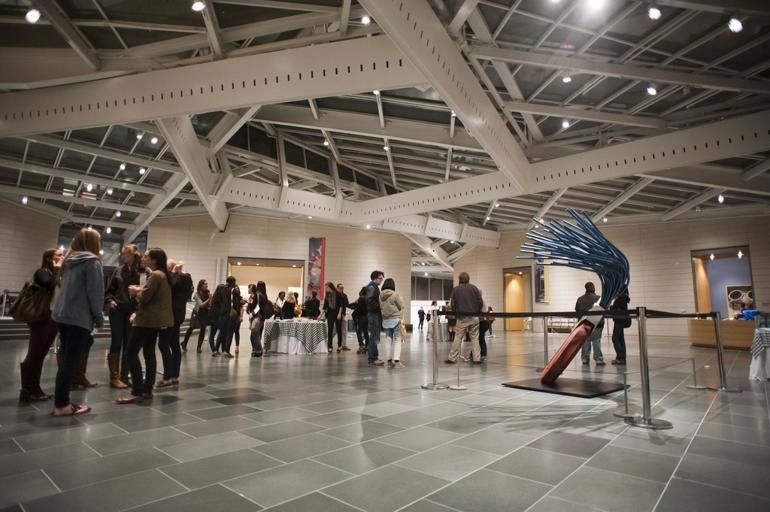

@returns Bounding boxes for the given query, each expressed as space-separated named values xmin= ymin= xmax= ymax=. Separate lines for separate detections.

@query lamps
xmin=647 ymin=3 xmax=662 ymax=21
xmin=647 ymin=83 xmax=657 ymax=95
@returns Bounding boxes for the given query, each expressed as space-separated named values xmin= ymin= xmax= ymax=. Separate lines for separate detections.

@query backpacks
xmin=264 ymin=295 xmax=274 ymax=318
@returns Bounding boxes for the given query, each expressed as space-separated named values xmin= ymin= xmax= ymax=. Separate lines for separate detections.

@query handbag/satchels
xmin=230 ymin=309 xmax=238 ymax=321
xmin=9 ymin=281 xmax=49 ymax=323
xmin=624 ymin=314 xmax=631 ymax=328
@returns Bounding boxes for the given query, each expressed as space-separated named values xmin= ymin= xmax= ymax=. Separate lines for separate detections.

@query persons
xmin=20 ymin=228 xmax=194 ymax=418
xmin=418 ymin=272 xmax=494 ymax=364
xmin=317 ymin=281 xmax=351 ymax=353
xmin=575 ymin=281 xmax=607 ymax=367
xmin=181 ymin=277 xmax=323 ymax=360
xmin=347 ymin=271 xmax=406 ymax=369
xmin=608 ymin=284 xmax=631 ymax=367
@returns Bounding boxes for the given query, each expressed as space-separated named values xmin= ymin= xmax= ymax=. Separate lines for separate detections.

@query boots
xmin=107 ymin=353 xmax=133 ymax=388
xmin=19 ymin=362 xmax=52 ymax=403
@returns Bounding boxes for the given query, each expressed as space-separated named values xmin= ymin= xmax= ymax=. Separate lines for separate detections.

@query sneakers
xmin=197 ymin=344 xmax=202 ymax=353
xmin=181 ymin=342 xmax=187 ymax=352
xmin=213 ymin=345 xmax=239 ymax=358
xmin=70 ymin=375 xmax=96 ymax=390
xmin=583 ymin=360 xmax=626 ymax=365
xmin=368 ymin=359 xmax=405 ymax=368
xmin=155 ymin=378 xmax=179 ymax=389
xmin=328 ymin=345 xmax=368 ymax=354
xmin=116 ymin=392 xmax=154 ymax=403
xmin=445 ymin=356 xmax=487 ymax=364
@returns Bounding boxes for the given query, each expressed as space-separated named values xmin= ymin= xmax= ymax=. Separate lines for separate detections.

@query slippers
xmin=52 ymin=403 xmax=90 ymax=416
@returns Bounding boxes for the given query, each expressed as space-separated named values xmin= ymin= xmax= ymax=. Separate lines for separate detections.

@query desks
xmin=263 ymin=319 xmax=328 ymax=355
xmin=430 ymin=323 xmax=467 ymax=342
xmin=748 ymin=328 xmax=770 ymax=381
